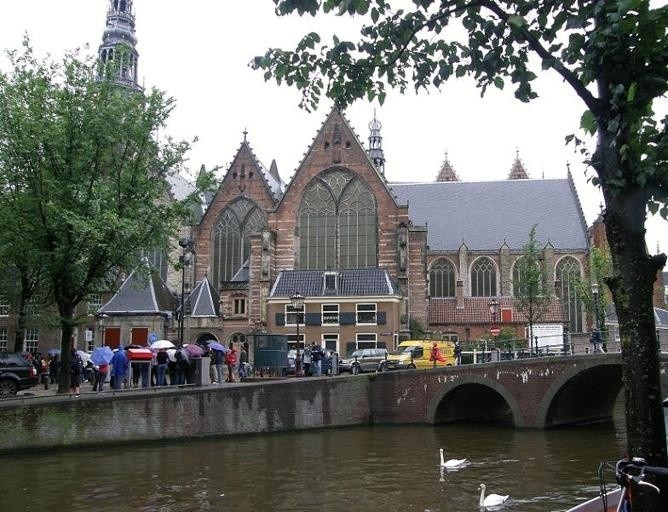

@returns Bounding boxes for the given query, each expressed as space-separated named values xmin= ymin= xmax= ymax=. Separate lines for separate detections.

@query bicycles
xmin=598 ymin=457 xmax=668 ymax=511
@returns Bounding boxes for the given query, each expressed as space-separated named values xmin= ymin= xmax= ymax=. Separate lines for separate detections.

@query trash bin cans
xmin=189 ymin=358 xmax=210 ymax=386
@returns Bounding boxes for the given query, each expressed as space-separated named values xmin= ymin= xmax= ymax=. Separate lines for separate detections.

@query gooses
xmin=440 ymin=449 xmax=471 ymax=467
xmin=477 ymin=483 xmax=509 ymax=507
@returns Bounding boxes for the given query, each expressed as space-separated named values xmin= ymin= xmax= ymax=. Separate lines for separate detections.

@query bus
xmin=460 ymin=351 xmax=573 ymax=365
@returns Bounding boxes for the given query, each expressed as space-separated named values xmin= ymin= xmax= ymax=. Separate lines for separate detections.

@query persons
xmin=453 ymin=341 xmax=462 ymax=365
xmin=33 ymin=344 xmax=241 ymax=391
xmin=311 ymin=341 xmax=325 ymax=378
xmin=304 ymin=343 xmax=313 ymax=376
xmin=429 ymin=341 xmax=446 ymax=368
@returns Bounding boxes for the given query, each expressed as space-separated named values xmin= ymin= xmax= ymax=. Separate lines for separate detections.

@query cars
xmin=0 ymin=351 xmax=42 ymax=399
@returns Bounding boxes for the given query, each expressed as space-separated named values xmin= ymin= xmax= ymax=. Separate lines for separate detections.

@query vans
xmin=286 ymin=339 xmax=455 ymax=375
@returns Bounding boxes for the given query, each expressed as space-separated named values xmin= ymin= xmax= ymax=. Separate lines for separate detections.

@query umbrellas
xmin=207 ymin=343 xmax=229 ymax=351
xmin=149 ymin=339 xmax=175 ymax=350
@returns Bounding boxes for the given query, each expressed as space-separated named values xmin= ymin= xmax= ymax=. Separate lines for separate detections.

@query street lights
xmin=488 ymin=298 xmax=500 ymax=349
xmin=290 ymin=292 xmax=305 ymax=376
xmin=588 ymin=283 xmax=600 ymax=329
xmin=179 ymin=238 xmax=196 ymax=348
xmin=97 ymin=313 xmax=109 ymax=347
